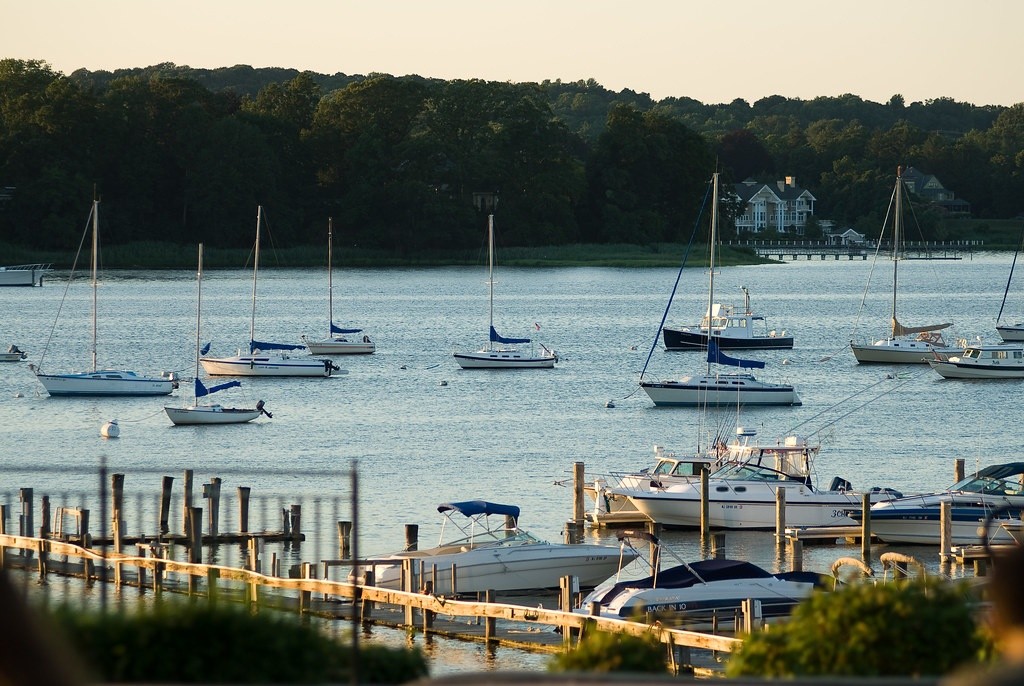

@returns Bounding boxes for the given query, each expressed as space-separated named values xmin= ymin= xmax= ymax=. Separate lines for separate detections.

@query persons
xmin=940 ymin=544 xmax=1024 ymax=686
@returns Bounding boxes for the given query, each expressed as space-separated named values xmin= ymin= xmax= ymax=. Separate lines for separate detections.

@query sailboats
xmin=637 ymin=171 xmax=803 ymax=405
xmin=849 ymin=165 xmax=982 ymax=363
xmin=303 ymin=216 xmax=377 ymax=354
xmin=198 ymin=205 xmax=329 ymax=377
xmin=453 ymin=215 xmax=556 ymax=369
xmin=162 ymin=243 xmax=264 ymax=423
xmin=995 ymin=225 xmax=1024 ymax=342
xmin=25 ymin=197 xmax=182 ymax=396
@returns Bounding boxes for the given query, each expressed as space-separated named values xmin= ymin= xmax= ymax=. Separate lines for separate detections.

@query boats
xmin=346 ymin=500 xmax=638 ymax=611
xmin=0 ymin=351 xmax=22 ymax=361
xmin=574 ymin=532 xmax=842 ymax=639
xmin=847 ymin=461 xmax=1024 ymax=545
xmin=663 ymin=287 xmax=795 ymax=351
xmin=0 ymin=264 xmax=52 ymax=286
xmin=556 ymin=429 xmax=902 ymax=540
xmin=928 ymin=343 xmax=1024 ymax=379
